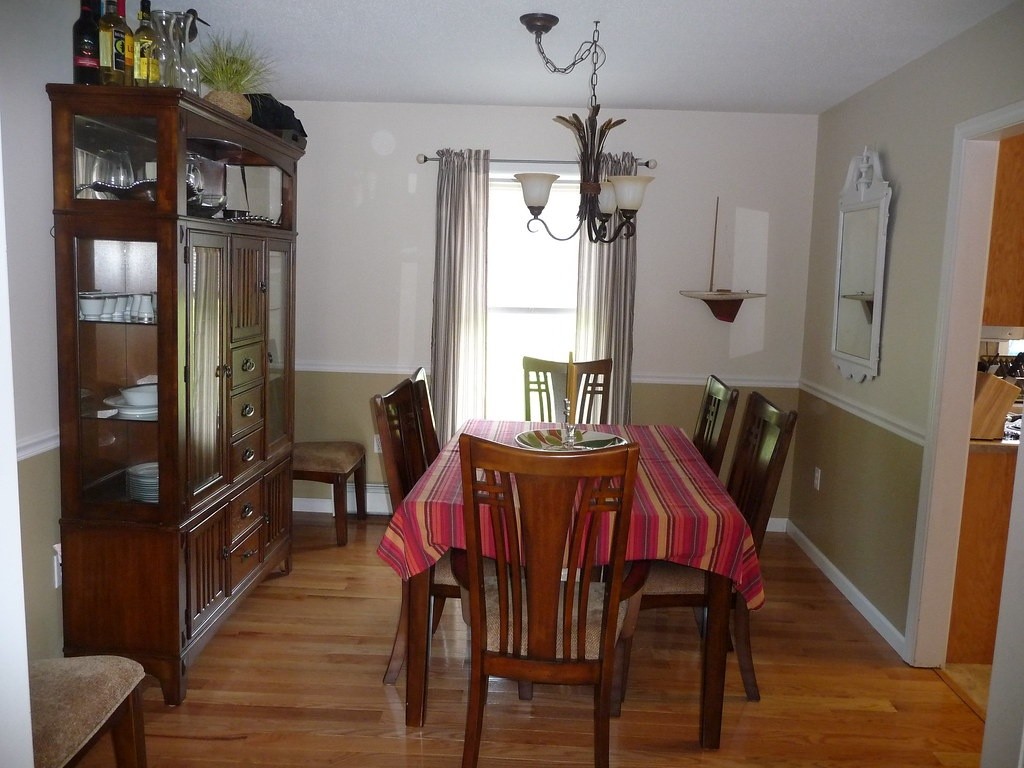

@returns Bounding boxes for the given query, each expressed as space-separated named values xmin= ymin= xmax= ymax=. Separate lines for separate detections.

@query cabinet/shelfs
xmin=45 ymin=81 xmax=306 ymax=709
xmin=979 ymin=134 xmax=1024 ymax=342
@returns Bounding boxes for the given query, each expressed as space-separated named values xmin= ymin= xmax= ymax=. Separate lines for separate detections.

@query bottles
xmin=150 ymin=10 xmax=181 ymax=88
xmin=171 ymin=11 xmax=201 ymax=97
xmin=72 ymin=0 xmax=160 ymax=87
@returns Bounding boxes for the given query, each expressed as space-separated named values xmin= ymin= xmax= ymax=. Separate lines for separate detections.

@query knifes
xmin=1004 ymin=352 xmax=1024 ymax=385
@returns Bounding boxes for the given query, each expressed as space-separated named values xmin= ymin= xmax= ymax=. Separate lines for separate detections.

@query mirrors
xmin=830 ymin=142 xmax=893 ymax=384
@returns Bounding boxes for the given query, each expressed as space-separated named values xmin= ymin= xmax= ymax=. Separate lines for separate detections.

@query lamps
xmin=514 ymin=11 xmax=658 ymax=245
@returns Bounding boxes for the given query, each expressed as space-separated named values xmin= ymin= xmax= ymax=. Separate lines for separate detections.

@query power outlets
xmin=373 ymin=434 xmax=384 ymax=454
xmin=53 ymin=554 xmax=62 ymax=589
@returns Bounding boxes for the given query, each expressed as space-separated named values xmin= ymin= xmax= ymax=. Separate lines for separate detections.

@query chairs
xmin=371 ymin=379 xmax=534 ymax=701
xmin=522 ymin=355 xmax=614 ymax=425
xmin=28 ymin=656 xmax=147 ymax=768
xmin=293 ymin=439 xmax=367 ymax=547
xmin=691 ymin=374 xmax=739 ymax=639
xmin=457 ymin=432 xmax=642 ymax=768
xmin=410 ymin=365 xmax=441 ymax=467
xmin=619 ymin=391 xmax=800 ymax=704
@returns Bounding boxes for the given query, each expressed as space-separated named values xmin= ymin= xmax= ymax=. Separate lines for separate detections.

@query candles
xmin=566 ymin=350 xmax=574 ymax=402
xmin=569 ymin=363 xmax=577 ymax=426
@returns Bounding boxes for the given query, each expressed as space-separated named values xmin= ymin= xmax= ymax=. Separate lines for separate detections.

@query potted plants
xmin=194 ymin=26 xmax=276 ymax=121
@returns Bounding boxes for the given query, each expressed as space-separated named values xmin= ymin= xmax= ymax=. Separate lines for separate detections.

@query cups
xmin=79 ymin=291 xmax=157 ymax=321
xmin=91 ymin=149 xmax=135 ymax=201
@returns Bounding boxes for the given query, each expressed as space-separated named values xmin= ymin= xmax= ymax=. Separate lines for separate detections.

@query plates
xmin=125 ymin=462 xmax=159 ymax=502
xmin=102 ymin=394 xmax=158 ymax=415
xmin=514 ymin=428 xmax=628 ymax=452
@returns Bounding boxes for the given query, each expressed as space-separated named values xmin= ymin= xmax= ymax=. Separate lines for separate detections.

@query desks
xmin=376 ymin=417 xmax=767 ymax=751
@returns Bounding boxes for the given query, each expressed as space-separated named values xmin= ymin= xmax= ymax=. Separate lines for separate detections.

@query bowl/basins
xmin=119 ymin=382 xmax=158 ymax=406
